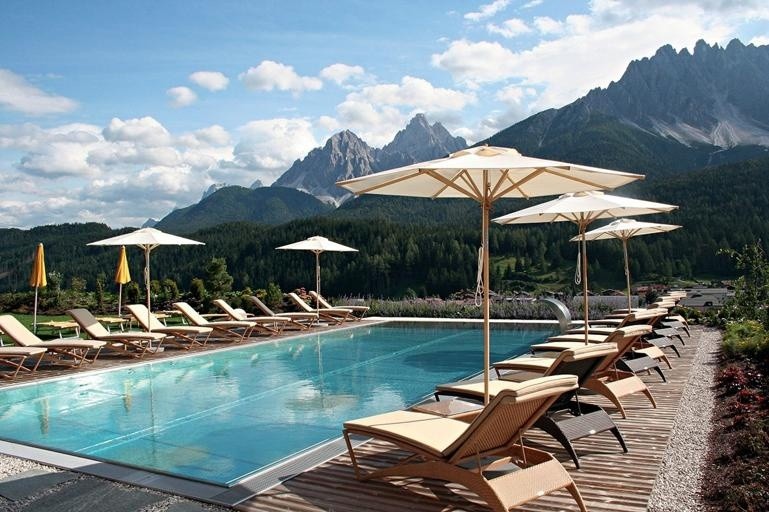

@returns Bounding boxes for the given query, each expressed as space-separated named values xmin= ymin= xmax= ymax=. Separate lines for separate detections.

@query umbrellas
xmin=275 ymin=234 xmax=359 ymax=328
xmin=116 ymin=244 xmax=133 ymax=319
xmin=84 ymin=226 xmax=205 ymax=356
xmin=30 ymin=243 xmax=47 ymax=335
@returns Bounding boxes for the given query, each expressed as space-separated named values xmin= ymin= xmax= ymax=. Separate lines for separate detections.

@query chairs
xmin=309 ymin=291 xmax=370 ymax=322
xmin=1 ymin=314 xmax=107 ymax=367
xmin=335 ymin=352 xmax=583 ymax=512
xmin=67 ymin=308 xmax=167 ymax=359
xmin=1 ymin=346 xmax=48 ymax=378
xmin=537 ymin=286 xmax=692 ymax=511
xmin=213 ymin=299 xmax=290 ymax=337
xmin=246 ymin=296 xmax=319 ymax=332
xmin=124 ymin=303 xmax=213 ymax=350
xmin=284 ymin=293 xmax=354 ymax=326
xmin=172 ymin=302 xmax=256 ymax=344
xmin=30 ymin=310 xmax=187 ymax=338
